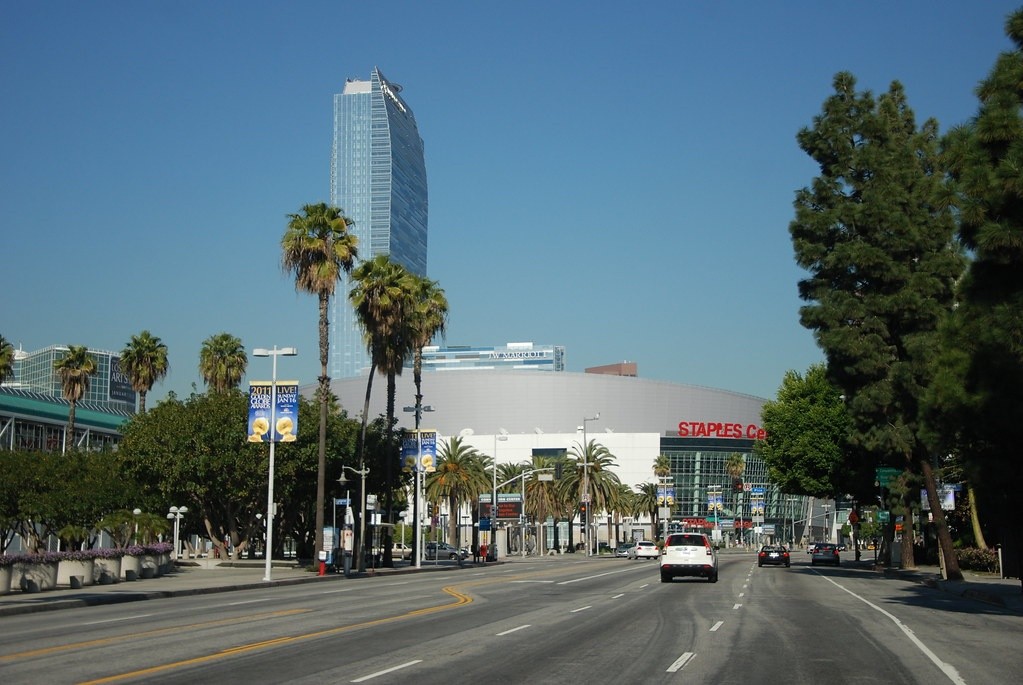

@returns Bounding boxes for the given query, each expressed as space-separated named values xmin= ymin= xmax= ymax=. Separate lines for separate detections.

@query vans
xmin=806 ymin=542 xmax=817 ymax=554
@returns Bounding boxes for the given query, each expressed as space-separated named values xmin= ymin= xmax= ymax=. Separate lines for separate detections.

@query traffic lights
xmin=732 ymin=478 xmax=743 ymax=494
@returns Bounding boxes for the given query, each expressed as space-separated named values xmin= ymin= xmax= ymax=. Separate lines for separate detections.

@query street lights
xmin=133 ymin=508 xmax=141 ymax=546
xmin=658 ymin=475 xmax=673 ymax=541
xmin=787 ymin=498 xmax=798 ymax=549
xmin=336 ymin=463 xmax=370 ymax=572
xmin=707 ymin=484 xmax=722 ymax=546
xmin=167 ymin=506 xmax=187 ymax=560
xmin=490 ymin=427 xmax=508 ymax=562
xmin=253 ymin=344 xmax=299 ymax=581
xmin=403 ymin=406 xmax=436 ymax=567
xmin=576 ymin=412 xmax=600 ymax=557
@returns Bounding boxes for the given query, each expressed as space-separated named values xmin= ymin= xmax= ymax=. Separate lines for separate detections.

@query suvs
xmin=660 ymin=532 xmax=718 ymax=583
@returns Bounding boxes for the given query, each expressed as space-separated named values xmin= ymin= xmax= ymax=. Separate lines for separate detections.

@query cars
xmin=758 ymin=546 xmax=790 ymax=567
xmin=379 ymin=542 xmax=415 ymax=560
xmin=627 ymin=541 xmax=659 ymax=559
xmin=420 ymin=541 xmax=468 ymax=560
xmin=617 ymin=543 xmax=634 ymax=556
xmin=812 ymin=543 xmax=840 ymax=565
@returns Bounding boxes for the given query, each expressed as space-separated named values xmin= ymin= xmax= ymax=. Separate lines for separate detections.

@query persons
xmin=471 ymin=541 xmax=497 ymax=562
